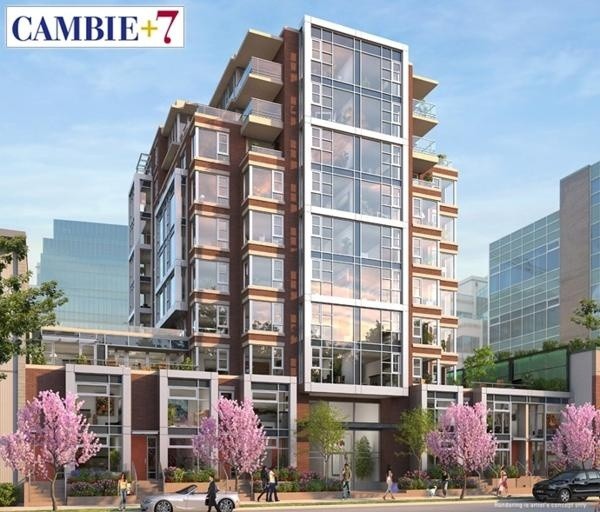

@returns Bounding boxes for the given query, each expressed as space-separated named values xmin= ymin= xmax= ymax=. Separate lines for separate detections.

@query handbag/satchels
xmin=206 ymin=499 xmax=214 ymax=505
xmin=215 ymin=485 xmax=220 ymax=493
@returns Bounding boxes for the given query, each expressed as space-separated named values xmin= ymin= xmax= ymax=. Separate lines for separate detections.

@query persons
xmin=256 ymin=464 xmax=269 ymax=501
xmin=382 ymin=463 xmax=396 ymax=499
xmin=497 ymin=465 xmax=511 ymax=498
xmin=205 ymin=473 xmax=221 ymax=512
xmin=341 ymin=463 xmax=353 ymax=498
xmin=441 ymin=471 xmax=450 ymax=497
xmin=267 ymin=465 xmax=280 ymax=502
xmin=117 ymin=472 xmax=129 ymax=511
